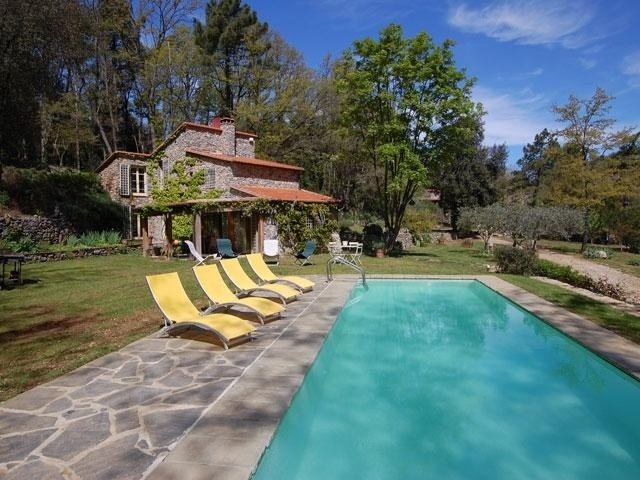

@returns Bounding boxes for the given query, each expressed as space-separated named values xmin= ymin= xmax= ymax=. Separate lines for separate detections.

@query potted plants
xmin=373 ymin=242 xmax=386 ymax=257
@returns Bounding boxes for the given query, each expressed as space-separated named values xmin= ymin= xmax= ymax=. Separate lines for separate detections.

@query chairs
xmin=144 ymin=238 xmax=316 ymax=351
xmin=328 ymin=240 xmax=364 ymax=269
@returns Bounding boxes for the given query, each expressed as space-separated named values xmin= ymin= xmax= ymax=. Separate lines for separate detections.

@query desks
xmin=0 ymin=254 xmax=26 ymax=285
